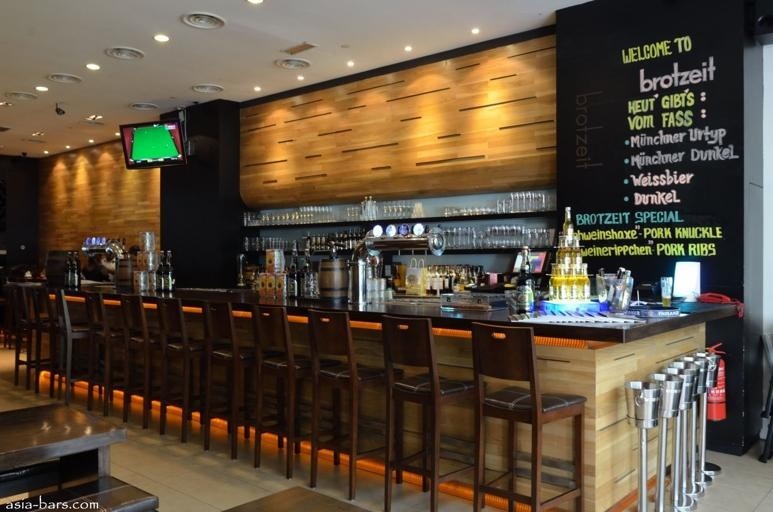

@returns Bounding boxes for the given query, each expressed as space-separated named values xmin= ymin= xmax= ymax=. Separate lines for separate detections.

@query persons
xmin=88 ymin=253 xmax=116 ymax=282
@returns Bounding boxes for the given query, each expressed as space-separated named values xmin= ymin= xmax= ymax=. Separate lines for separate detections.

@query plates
xmin=372 ymin=223 xmax=428 ymax=239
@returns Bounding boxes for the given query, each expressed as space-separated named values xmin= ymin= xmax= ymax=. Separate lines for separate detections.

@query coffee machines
xmin=79 ymin=239 xmax=127 ymax=286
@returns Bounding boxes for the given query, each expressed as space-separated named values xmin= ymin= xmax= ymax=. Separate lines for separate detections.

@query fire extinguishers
xmin=705 ymin=343 xmax=726 ymax=421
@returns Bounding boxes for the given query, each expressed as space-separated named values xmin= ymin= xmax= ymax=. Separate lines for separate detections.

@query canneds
xmin=133 ymin=232 xmax=156 ymax=292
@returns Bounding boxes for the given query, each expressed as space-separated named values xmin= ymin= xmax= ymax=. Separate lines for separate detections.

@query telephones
xmin=696 ymin=293 xmax=731 ymax=304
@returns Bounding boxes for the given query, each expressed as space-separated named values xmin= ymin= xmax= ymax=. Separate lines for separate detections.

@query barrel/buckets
xmin=318 ymin=258 xmax=350 ymax=307
xmin=115 ymin=252 xmax=133 ymax=293
xmin=623 ymin=349 xmax=721 ymax=430
xmin=46 ymin=249 xmax=66 ymax=289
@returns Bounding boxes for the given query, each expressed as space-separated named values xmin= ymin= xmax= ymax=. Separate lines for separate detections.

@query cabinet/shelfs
xmin=243 ymin=187 xmax=557 ymax=292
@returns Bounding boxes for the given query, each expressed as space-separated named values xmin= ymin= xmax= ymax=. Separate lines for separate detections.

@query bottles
xmin=243 ymin=229 xmax=368 ymax=250
xmin=327 ymin=244 xmax=337 ymax=260
xmin=547 ymin=206 xmax=591 ymax=300
xmin=287 ymin=249 xmax=319 ymax=298
xmin=242 ymin=195 xmax=416 ymax=224
xmin=598 ymin=267 xmax=630 ymax=308
xmin=424 ymin=264 xmax=487 ymax=297
xmin=64 ymin=251 xmax=80 ymax=287
xmin=133 ymin=230 xmax=175 ymax=293
xmin=517 ymin=246 xmax=535 ymax=312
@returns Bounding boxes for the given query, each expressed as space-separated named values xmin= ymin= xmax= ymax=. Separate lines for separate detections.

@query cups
xmin=660 ymin=276 xmax=673 ymax=307
xmin=442 ymin=189 xmax=550 ymax=249
xmin=344 ymin=257 xmax=393 ymax=305
xmin=595 ymin=274 xmax=633 ymax=315
xmin=256 ymin=272 xmax=286 ymax=300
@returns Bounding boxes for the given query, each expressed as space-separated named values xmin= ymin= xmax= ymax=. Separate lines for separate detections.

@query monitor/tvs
xmin=119 ymin=118 xmax=188 ymax=170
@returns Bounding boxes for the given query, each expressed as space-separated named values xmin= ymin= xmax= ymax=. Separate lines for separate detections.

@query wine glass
xmin=622 ymin=351 xmax=722 ymax=512
